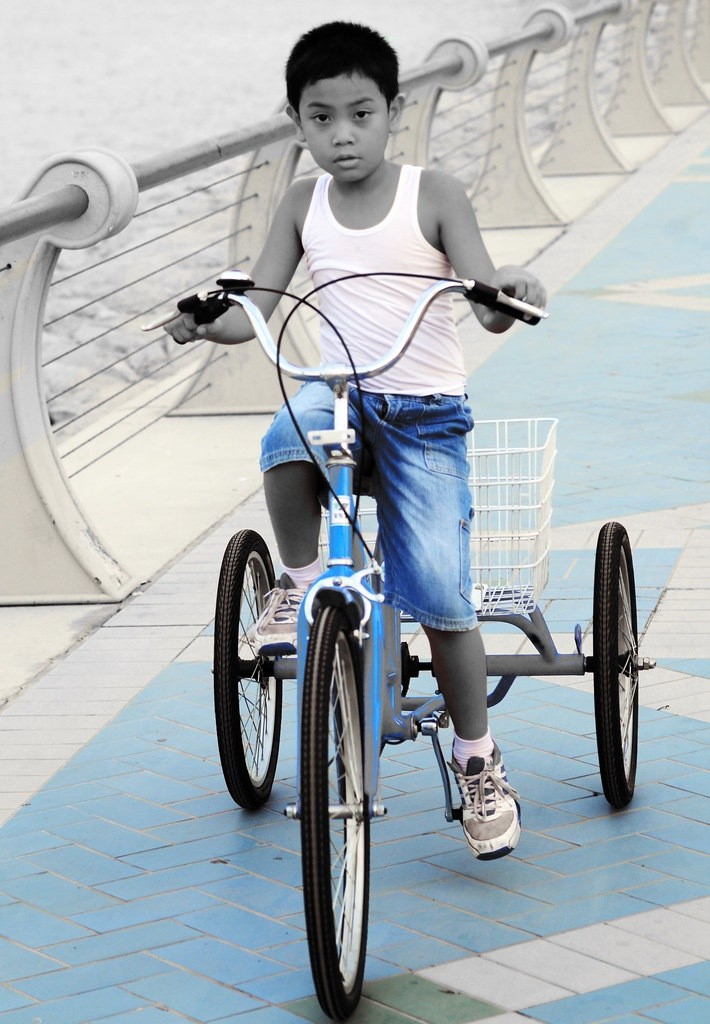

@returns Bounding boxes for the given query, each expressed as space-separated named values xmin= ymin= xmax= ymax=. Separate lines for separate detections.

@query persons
xmin=160 ymin=22 xmax=552 ymax=864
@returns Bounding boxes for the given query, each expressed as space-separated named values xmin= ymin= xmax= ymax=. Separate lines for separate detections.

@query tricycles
xmin=140 ymin=273 xmax=657 ymax=1024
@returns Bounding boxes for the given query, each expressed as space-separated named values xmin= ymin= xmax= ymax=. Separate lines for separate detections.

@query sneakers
xmin=447 ymin=740 xmax=523 ymax=861
xmin=251 ymin=574 xmax=306 ymax=657
